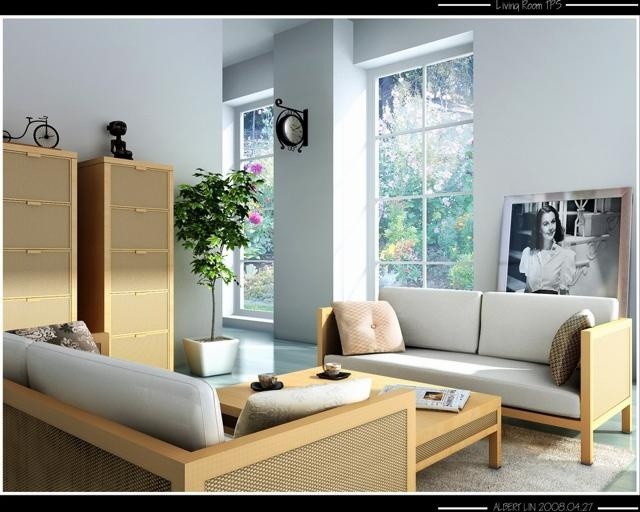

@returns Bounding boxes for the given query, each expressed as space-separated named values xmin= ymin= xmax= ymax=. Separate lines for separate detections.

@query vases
xmin=183 ymin=334 xmax=241 ymax=378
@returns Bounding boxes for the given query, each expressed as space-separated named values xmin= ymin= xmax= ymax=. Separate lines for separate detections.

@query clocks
xmin=275 ymin=97 xmax=309 ymax=153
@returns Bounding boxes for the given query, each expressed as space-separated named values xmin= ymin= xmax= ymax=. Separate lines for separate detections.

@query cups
xmin=257 ymin=362 xmax=342 ymax=386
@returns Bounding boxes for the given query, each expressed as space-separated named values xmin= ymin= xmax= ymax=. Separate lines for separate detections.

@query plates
xmin=251 ymin=371 xmax=351 ymax=393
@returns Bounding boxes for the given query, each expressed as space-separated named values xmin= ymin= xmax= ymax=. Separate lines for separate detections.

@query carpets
xmin=416 ymin=424 xmax=635 ymax=493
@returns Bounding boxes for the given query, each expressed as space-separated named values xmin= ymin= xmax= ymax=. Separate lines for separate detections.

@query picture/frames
xmin=496 ymin=187 xmax=632 ymax=318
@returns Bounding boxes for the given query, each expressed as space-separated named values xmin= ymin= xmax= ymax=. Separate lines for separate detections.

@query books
xmin=377 ymin=382 xmax=473 ymax=414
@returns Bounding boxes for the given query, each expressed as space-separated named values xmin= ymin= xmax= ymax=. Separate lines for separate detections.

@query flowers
xmin=174 ymin=161 xmax=266 ymax=341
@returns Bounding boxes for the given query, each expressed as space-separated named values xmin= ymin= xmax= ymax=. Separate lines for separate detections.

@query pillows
xmin=8 ymin=320 xmax=102 ymax=356
xmin=549 ymin=309 xmax=596 ymax=387
xmin=231 ymin=378 xmax=372 ymax=439
xmin=329 ymin=300 xmax=406 ymax=357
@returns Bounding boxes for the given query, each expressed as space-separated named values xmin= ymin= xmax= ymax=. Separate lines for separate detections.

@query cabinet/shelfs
xmin=78 ymin=156 xmax=175 ymax=372
xmin=3 ymin=141 xmax=77 ymax=332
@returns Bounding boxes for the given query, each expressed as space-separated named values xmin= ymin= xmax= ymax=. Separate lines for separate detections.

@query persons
xmin=517 ymin=206 xmax=577 ymax=295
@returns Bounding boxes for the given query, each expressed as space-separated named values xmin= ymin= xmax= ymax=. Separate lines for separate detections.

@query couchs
xmin=5 ymin=319 xmax=416 ymax=492
xmin=316 ymin=286 xmax=635 ymax=466
